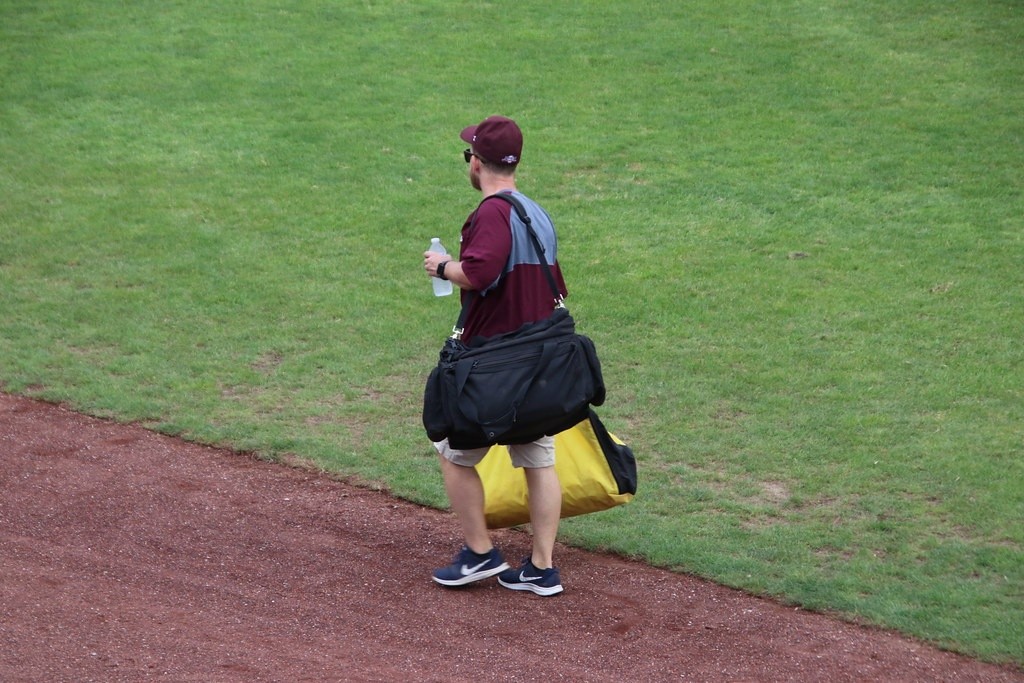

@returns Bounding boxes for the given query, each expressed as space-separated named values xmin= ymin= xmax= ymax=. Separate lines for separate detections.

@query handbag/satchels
xmin=422 ymin=308 xmax=607 ymax=454
xmin=473 ymin=410 xmax=638 ymax=529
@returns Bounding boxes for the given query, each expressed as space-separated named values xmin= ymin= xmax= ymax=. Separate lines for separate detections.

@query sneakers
xmin=497 ymin=556 xmax=564 ymax=596
xmin=432 ymin=545 xmax=511 ymax=586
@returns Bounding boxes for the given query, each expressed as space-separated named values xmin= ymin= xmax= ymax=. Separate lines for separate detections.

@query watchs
xmin=437 ymin=261 xmax=449 ymax=281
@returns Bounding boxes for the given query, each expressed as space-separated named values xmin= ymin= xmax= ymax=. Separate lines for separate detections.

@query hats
xmin=460 ymin=116 xmax=523 ymax=165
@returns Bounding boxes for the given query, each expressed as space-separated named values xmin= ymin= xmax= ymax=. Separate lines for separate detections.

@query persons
xmin=425 ymin=114 xmax=571 ymax=597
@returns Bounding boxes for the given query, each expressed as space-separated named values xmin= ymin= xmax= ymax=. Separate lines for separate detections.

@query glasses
xmin=464 ymin=149 xmax=486 ymax=164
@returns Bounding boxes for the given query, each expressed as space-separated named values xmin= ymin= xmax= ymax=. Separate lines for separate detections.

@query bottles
xmin=430 ymin=238 xmax=453 ymax=298
